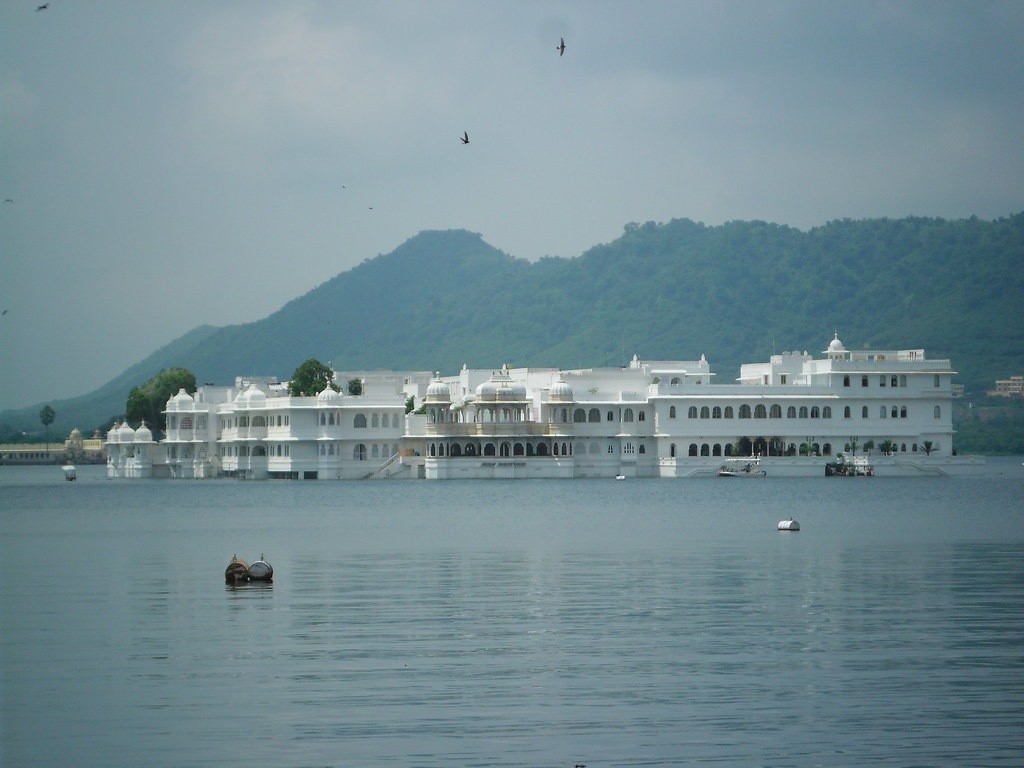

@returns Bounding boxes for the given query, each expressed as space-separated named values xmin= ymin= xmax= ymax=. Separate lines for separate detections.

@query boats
xmin=719 ymin=452 xmax=766 ymax=478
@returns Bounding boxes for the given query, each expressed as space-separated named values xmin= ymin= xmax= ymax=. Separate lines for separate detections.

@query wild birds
xmin=339 ymin=184 xmax=350 ymax=188
xmin=4 ymin=198 xmax=14 ymax=205
xmin=34 ymin=3 xmax=49 ymax=13
xmin=459 ymin=131 xmax=470 ymax=144
xmin=556 ymin=37 xmax=566 ymax=56
xmin=1 ymin=310 xmax=8 ymax=315
